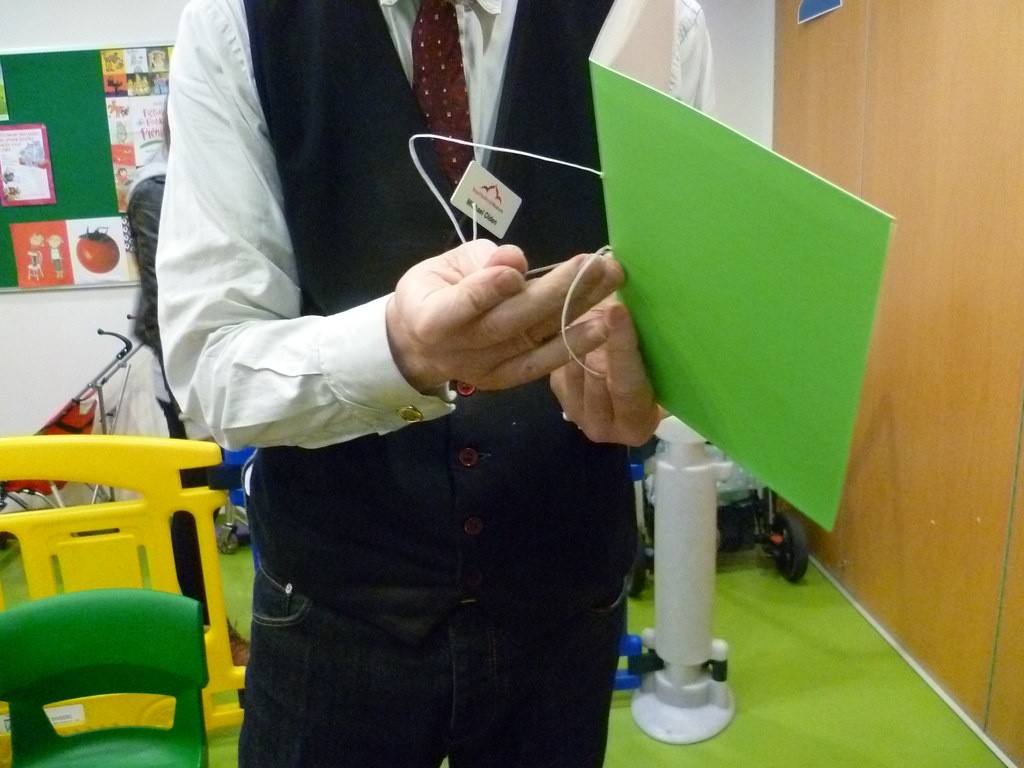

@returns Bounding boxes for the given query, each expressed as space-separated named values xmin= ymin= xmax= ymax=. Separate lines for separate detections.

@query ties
xmin=412 ymin=1 xmax=476 ymax=195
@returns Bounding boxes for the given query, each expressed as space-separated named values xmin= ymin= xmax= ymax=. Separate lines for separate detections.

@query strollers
xmin=0 ymin=314 xmax=149 ymax=550
xmin=632 ymin=420 xmax=809 ymax=595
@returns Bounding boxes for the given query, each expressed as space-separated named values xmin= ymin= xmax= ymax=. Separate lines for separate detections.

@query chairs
xmin=0 ymin=588 xmax=209 ymax=767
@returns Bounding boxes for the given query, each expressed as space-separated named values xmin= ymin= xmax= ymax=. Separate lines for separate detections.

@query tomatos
xmin=76 ymin=226 xmax=120 ymax=274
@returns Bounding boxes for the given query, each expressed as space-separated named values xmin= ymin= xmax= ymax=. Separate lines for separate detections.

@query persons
xmin=127 ymin=92 xmax=244 ymax=490
xmin=156 ymin=0 xmax=712 ymax=767
